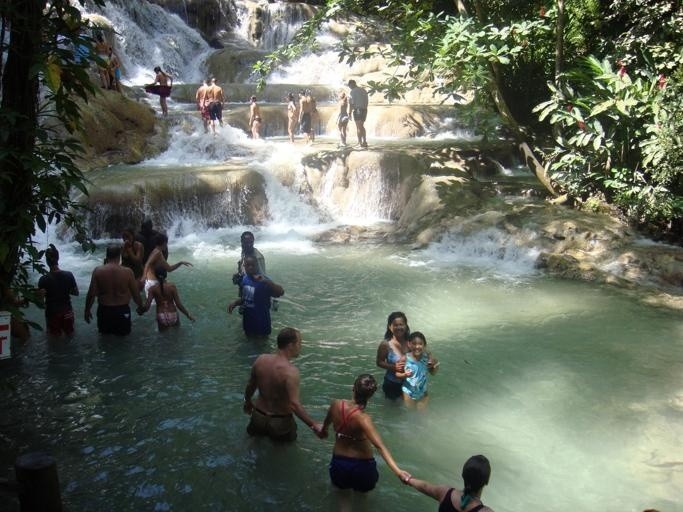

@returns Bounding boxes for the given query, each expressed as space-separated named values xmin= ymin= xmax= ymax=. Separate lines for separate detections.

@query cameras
xmin=238 ymin=304 xmax=247 ymax=314
xmin=232 ymin=274 xmax=243 ymax=284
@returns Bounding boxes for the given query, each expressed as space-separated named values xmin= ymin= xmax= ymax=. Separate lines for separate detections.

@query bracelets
xmin=404 ymin=476 xmax=411 ymax=485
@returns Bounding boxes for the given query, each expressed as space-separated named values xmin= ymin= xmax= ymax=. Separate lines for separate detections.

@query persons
xmin=232 ymin=231 xmax=266 ymax=297
xmin=400 ymin=453 xmax=494 ymax=512
xmin=376 ymin=311 xmax=440 ymax=401
xmin=93 ymin=38 xmax=369 ymax=147
xmin=227 ymin=255 xmax=285 ymax=340
xmin=242 ymin=327 xmax=323 ymax=443
xmin=319 ymin=372 xmax=411 ymax=512
xmin=38 ymin=219 xmax=195 ymax=337
xmin=392 ymin=332 xmax=436 ymax=411
